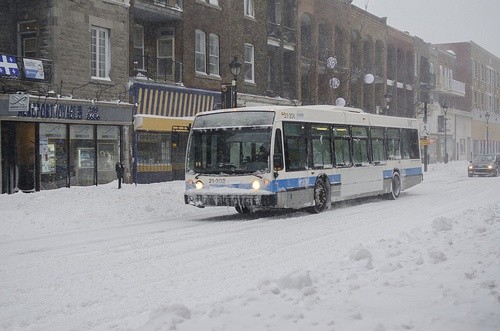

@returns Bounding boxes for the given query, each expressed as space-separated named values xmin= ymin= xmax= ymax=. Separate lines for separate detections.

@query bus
xmin=182 ymin=104 xmax=425 ymax=215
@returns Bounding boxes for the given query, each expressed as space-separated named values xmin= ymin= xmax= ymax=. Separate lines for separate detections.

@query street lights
xmin=485 ymin=111 xmax=490 ymax=154
xmin=442 ymin=102 xmax=449 ymax=163
xmin=228 ymin=55 xmax=243 ymax=108
xmin=384 ymin=89 xmax=393 ymax=115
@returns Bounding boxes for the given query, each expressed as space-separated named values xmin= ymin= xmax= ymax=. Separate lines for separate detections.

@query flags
xmin=0 ymin=55 xmax=20 ymax=75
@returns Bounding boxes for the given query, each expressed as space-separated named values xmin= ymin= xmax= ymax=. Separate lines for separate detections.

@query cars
xmin=467 ymin=152 xmax=500 ymax=177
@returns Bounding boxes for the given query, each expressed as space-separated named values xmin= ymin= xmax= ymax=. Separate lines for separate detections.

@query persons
xmin=258 ymin=145 xmax=268 ymax=162
xmin=115 ymin=162 xmax=124 ymax=189
xmin=36 ymin=64 xmax=44 ymax=79
